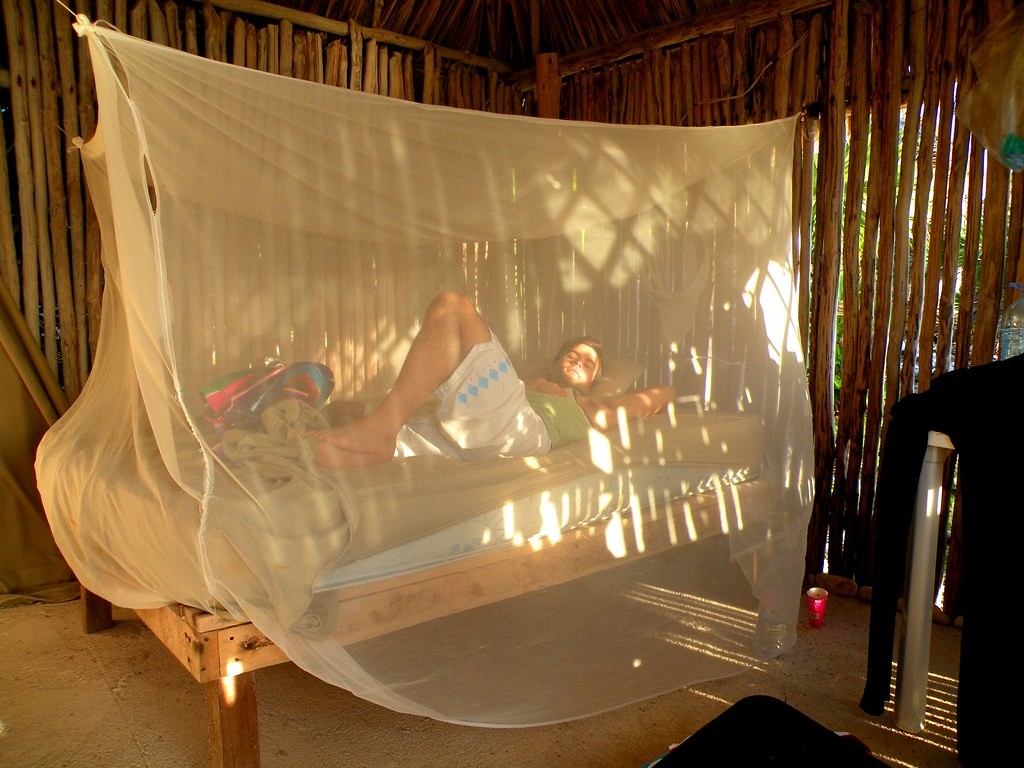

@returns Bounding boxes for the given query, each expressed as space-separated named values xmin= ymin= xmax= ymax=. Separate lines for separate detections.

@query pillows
xmin=516 ymin=338 xmax=644 ymax=400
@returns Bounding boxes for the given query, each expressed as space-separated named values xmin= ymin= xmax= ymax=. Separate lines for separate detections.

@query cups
xmin=806 ymin=587 xmax=828 ymax=628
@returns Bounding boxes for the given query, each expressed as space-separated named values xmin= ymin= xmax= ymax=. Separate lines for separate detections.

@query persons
xmin=306 ymin=291 xmax=676 ymax=471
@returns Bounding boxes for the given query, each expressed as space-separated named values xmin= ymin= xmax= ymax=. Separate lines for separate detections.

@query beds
xmin=34 ymin=398 xmax=816 ymax=768
